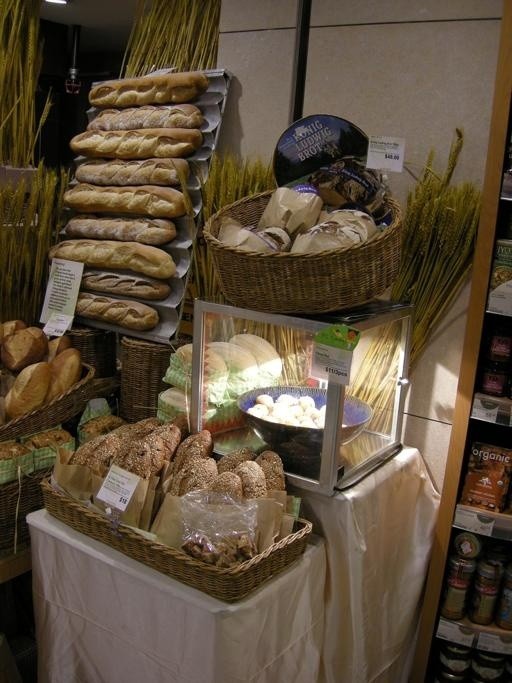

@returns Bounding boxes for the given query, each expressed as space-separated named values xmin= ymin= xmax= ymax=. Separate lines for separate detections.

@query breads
xmin=74 ymin=270 xmax=171 ymax=330
xmin=65 ymin=214 xmax=178 ymax=246
xmin=86 ymin=103 xmax=206 ymax=130
xmin=24 ymin=428 xmax=71 ymax=449
xmin=87 ymin=71 xmax=210 ymax=107
xmin=160 ymin=333 xmax=283 ymax=412
xmin=67 ymin=416 xmax=214 ymax=479
xmin=170 ymin=440 xmax=286 ymax=567
xmin=1 ymin=319 xmax=82 ymax=427
xmin=62 ymin=182 xmax=187 ymax=219
xmin=74 ymin=157 xmax=190 ymax=186
xmin=80 ymin=415 xmax=129 ymax=439
xmin=1 ymin=441 xmax=31 ymax=460
xmin=69 ymin=127 xmax=203 ymax=157
xmin=47 ymin=238 xmax=177 ymax=280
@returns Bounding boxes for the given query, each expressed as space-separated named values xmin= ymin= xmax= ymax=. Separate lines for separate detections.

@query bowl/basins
xmin=237 ymin=387 xmax=374 ymax=460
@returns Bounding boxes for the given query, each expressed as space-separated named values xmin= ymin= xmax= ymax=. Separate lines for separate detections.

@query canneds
xmin=441 ymin=532 xmax=512 ymax=631
xmin=433 ymin=644 xmax=512 ymax=682
xmin=479 ymin=325 xmax=512 ymax=400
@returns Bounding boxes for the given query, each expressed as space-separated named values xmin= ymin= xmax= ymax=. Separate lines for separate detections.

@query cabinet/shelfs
xmin=407 ymin=6 xmax=512 ymax=680
xmin=0 ymin=542 xmax=37 ymax=682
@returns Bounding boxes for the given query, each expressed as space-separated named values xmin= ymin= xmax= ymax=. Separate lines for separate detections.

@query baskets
xmin=0 ymin=469 xmax=53 ymax=560
xmin=64 ymin=328 xmax=117 ymax=405
xmin=1 ymin=360 xmax=96 ymax=445
xmin=117 ymin=332 xmax=192 ymax=423
xmin=202 ymin=187 xmax=405 ymax=315
xmin=40 ymin=467 xmax=313 ymax=604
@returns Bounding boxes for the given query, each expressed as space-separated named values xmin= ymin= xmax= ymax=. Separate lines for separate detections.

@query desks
xmin=285 ymin=448 xmax=441 ymax=683
xmin=23 ymin=505 xmax=327 ymax=683
